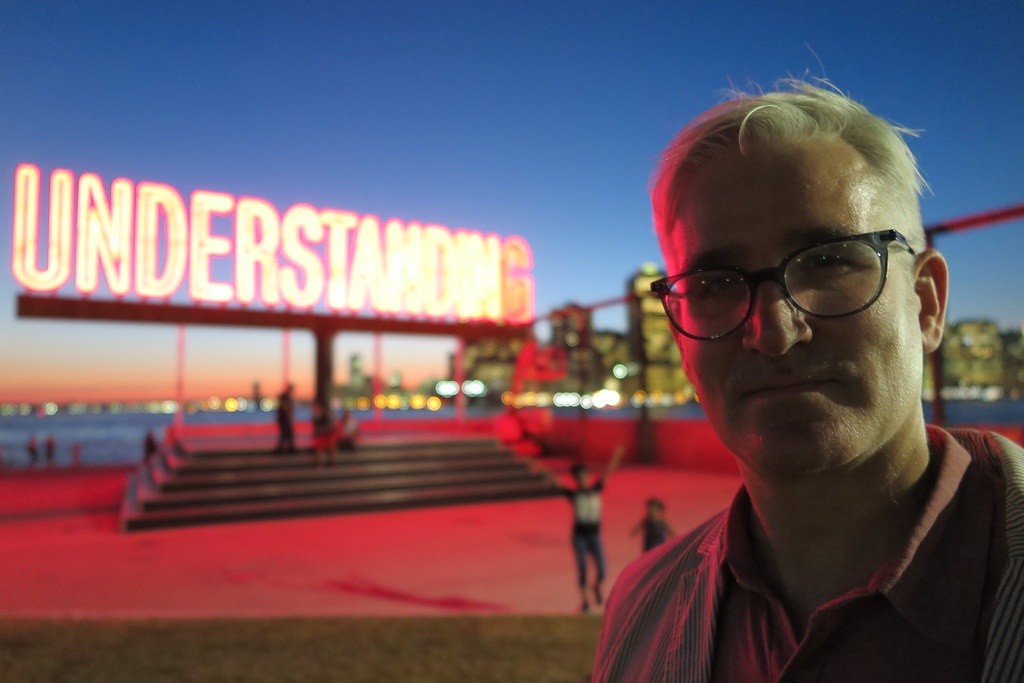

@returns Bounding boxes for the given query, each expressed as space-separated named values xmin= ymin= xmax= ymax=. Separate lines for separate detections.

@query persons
xmin=310 ymin=383 xmax=337 ymax=468
xmin=25 ymin=433 xmax=38 ymax=471
xmin=592 ymin=74 xmax=1024 ymax=683
xmin=143 ymin=426 xmax=158 ymax=468
xmin=539 ymin=446 xmax=625 ymax=613
xmin=336 ymin=408 xmax=360 ymax=454
xmin=42 ymin=433 xmax=56 ymax=472
xmin=68 ymin=437 xmax=81 ymax=472
xmin=630 ymin=497 xmax=676 ymax=552
xmin=275 ymin=380 xmax=298 ymax=453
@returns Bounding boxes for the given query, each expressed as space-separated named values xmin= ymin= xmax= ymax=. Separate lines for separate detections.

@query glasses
xmin=649 ymin=229 xmax=915 ymax=341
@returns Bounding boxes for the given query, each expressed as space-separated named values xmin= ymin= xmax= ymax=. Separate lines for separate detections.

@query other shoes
xmin=581 ymin=602 xmax=588 ymax=610
xmin=591 ymin=584 xmax=604 ymax=603
